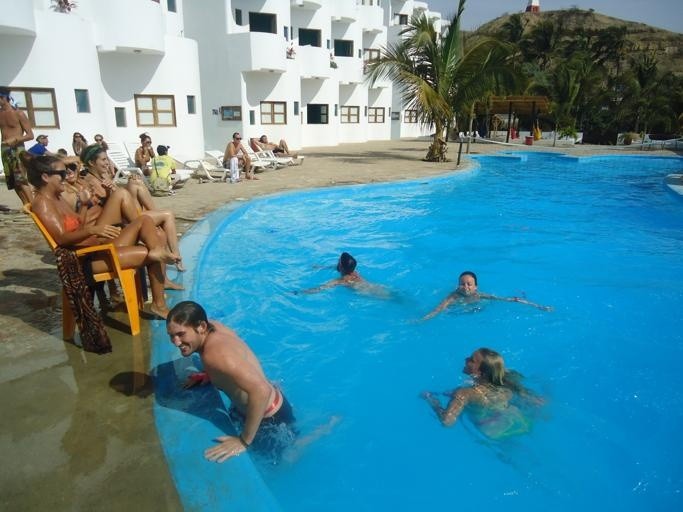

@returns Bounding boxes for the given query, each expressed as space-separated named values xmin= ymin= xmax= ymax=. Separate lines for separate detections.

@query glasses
xmin=47 ymin=169 xmax=67 ymax=180
xmin=74 ymin=135 xmax=82 ymax=138
xmin=64 ymin=163 xmax=77 ymax=172
xmin=235 ymin=137 xmax=242 ymax=141
xmin=143 ymin=140 xmax=151 ymax=144
xmin=95 ymin=138 xmax=102 ymax=141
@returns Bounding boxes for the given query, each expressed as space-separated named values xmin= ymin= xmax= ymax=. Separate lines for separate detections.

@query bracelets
xmin=239 ymin=434 xmax=250 ymax=448
xmin=14 ymin=136 xmax=17 ymax=145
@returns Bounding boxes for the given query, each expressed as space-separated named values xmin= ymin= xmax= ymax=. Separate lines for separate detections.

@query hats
xmin=36 ymin=135 xmax=48 ymax=141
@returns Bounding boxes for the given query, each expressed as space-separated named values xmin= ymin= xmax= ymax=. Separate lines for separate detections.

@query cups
xmin=77 ymin=188 xmax=92 ymax=207
xmin=250 ymin=175 xmax=253 ymax=181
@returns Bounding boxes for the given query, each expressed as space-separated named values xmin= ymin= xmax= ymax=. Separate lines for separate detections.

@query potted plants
xmin=616 ymin=132 xmax=639 ymax=145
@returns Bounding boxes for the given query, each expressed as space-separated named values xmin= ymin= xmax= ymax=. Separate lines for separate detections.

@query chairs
xmin=167 ymin=138 xmax=304 ymax=182
xmin=106 ymin=141 xmax=163 ymax=192
xmin=21 ymin=203 xmax=141 ymax=337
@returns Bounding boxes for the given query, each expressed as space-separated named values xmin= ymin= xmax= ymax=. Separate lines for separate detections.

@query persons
xmin=421 ymin=348 xmax=544 ymax=442
xmin=222 ymin=133 xmax=256 ymax=182
xmin=19 ymin=132 xmax=187 ymax=321
xmin=254 ymin=135 xmax=298 ymax=155
xmin=167 ymin=300 xmax=340 ymax=477
xmin=0 ymin=86 xmax=34 ymax=206
xmin=285 ymin=252 xmax=401 ymax=301
xmin=419 ymin=271 xmax=552 ymax=320
